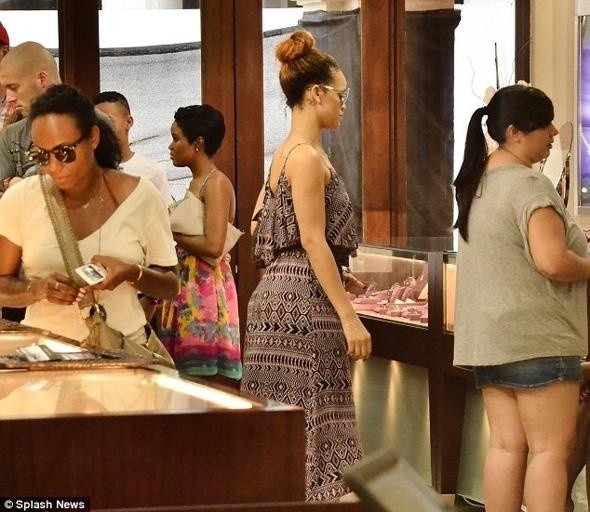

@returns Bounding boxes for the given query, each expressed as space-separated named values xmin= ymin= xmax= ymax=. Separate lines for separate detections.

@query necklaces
xmin=64 ymin=176 xmax=109 ymax=215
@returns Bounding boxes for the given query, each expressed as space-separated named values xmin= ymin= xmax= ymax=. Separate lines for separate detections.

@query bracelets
xmin=125 ymin=263 xmax=142 ymax=285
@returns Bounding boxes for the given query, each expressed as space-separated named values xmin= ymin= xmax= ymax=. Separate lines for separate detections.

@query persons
xmin=1 ymin=81 xmax=178 ymax=355
xmin=451 ymin=84 xmax=590 ymax=512
xmin=153 ymin=102 xmax=242 ymax=389
xmin=243 ymin=27 xmax=373 ymax=502
xmin=0 ymin=40 xmax=114 ymax=200
xmin=0 ymin=23 xmax=21 ymax=130
xmin=90 ymin=91 xmax=173 ymax=324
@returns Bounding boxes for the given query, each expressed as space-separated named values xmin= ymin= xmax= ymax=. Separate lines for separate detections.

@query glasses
xmin=309 ymin=85 xmax=351 ymax=105
xmin=27 ymin=134 xmax=86 ymax=167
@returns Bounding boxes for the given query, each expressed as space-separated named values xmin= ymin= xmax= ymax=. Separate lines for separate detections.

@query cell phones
xmin=74 ymin=263 xmax=107 ymax=287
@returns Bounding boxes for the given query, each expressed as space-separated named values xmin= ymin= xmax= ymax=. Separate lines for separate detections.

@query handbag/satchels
xmin=81 ymin=326 xmax=176 ymax=373
xmin=169 ymin=191 xmax=243 ymax=269
